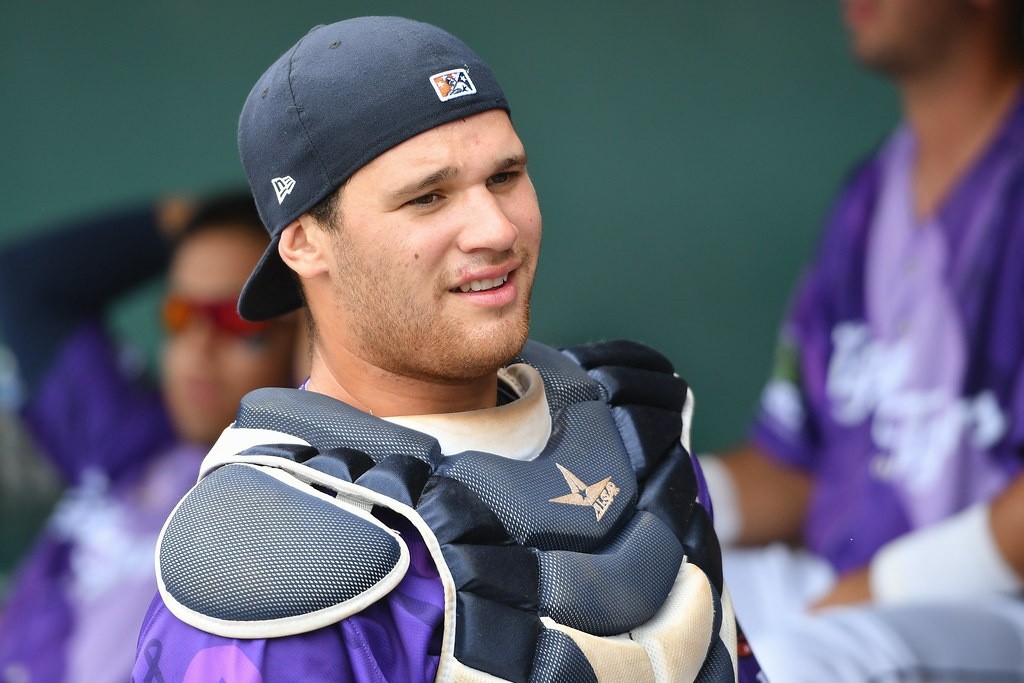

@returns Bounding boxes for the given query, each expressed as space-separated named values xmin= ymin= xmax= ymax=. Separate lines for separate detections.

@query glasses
xmin=158 ymin=295 xmax=284 ymax=342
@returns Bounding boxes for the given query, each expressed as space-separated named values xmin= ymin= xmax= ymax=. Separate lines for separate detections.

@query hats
xmin=236 ymin=14 xmax=511 ymax=323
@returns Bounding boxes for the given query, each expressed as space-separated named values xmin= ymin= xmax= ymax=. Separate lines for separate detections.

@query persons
xmin=0 ymin=188 xmax=310 ymax=683
xmin=698 ymin=0 xmax=1024 ymax=683
xmin=127 ymin=14 xmax=769 ymax=683
